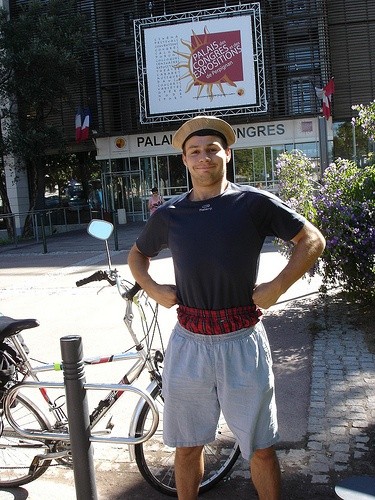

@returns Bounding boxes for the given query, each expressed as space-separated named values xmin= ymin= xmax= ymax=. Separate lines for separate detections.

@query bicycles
xmin=0 ymin=217 xmax=241 ymax=498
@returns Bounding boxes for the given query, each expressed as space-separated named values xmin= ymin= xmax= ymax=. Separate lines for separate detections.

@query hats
xmin=172 ymin=115 xmax=237 ymax=149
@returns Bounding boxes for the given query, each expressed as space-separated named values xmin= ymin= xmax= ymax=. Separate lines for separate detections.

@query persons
xmin=127 ymin=116 xmax=327 ymax=500
xmin=149 ymin=187 xmax=163 ymax=215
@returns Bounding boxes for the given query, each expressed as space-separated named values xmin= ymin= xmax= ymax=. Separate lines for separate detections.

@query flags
xmin=315 ymin=79 xmax=334 ymax=100
xmin=322 ymin=97 xmax=332 ymax=120
xmin=74 ymin=110 xmax=90 ymax=144
